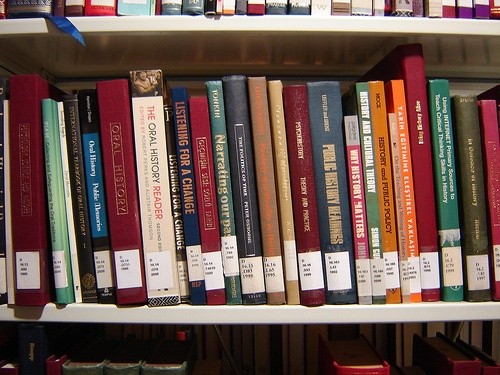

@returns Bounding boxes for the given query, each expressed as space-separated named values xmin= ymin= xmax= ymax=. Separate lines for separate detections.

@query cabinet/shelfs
xmin=0 ymin=17 xmax=500 ymax=324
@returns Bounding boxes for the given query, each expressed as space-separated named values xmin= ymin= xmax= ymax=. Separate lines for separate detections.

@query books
xmin=1 ymin=43 xmax=500 ymax=307
xmin=1 ymin=0 xmax=500 ymax=20
xmin=1 ymin=330 xmax=500 ymax=375
